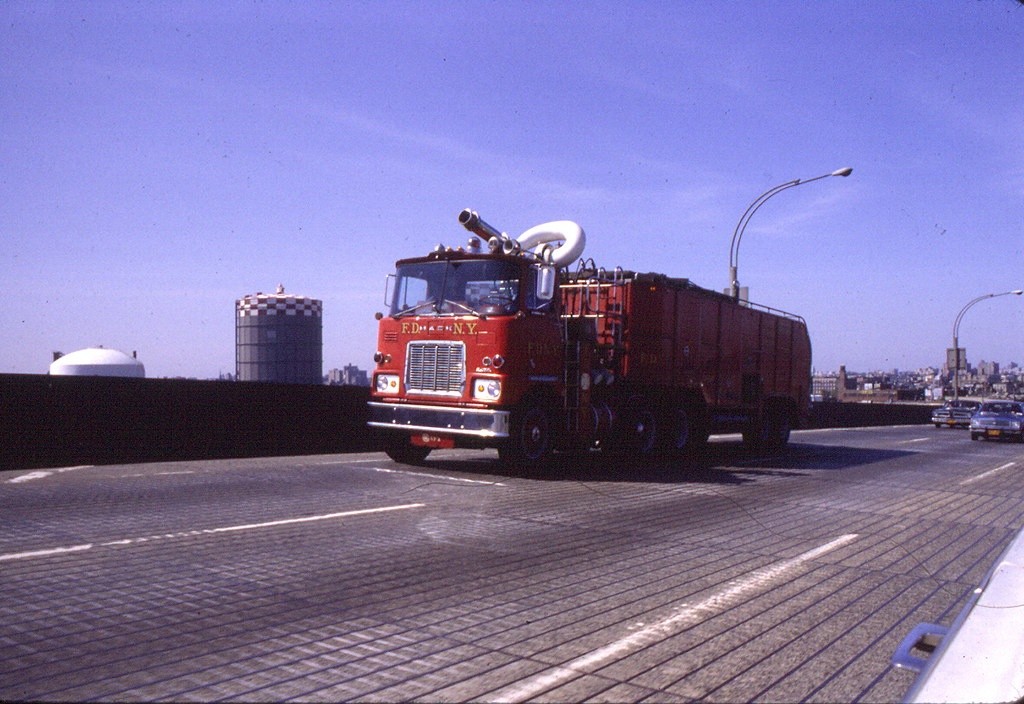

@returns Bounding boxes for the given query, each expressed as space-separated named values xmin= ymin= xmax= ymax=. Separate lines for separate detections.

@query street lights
xmin=950 ymin=290 xmax=1023 ymax=405
xmin=727 ymin=167 xmax=855 ymax=309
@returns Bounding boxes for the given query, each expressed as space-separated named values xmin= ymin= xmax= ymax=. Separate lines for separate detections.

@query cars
xmin=970 ymin=400 xmax=1024 ymax=441
xmin=932 ymin=400 xmax=982 ymax=429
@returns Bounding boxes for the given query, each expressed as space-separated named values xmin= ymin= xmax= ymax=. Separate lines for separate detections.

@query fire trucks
xmin=362 ymin=208 xmax=818 ymax=479
